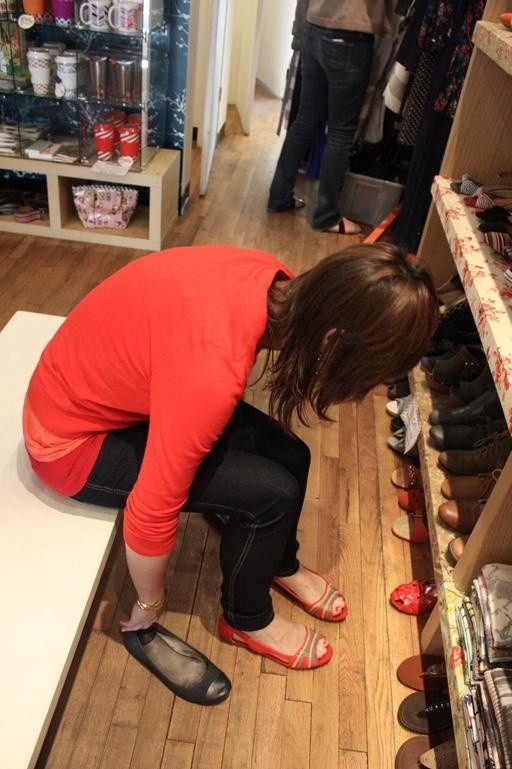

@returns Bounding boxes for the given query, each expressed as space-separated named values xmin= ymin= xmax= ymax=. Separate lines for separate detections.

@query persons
xmin=267 ymin=0 xmax=384 ymax=236
xmin=23 ymin=241 xmax=440 ymax=671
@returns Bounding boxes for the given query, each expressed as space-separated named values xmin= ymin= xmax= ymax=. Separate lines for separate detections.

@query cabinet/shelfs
xmin=408 ymin=2 xmax=512 ymax=769
xmin=1 ymin=0 xmax=181 ymax=252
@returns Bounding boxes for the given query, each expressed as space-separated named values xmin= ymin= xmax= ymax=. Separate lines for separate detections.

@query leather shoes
xmin=327 ymin=217 xmax=362 ymax=233
xmin=123 ymin=621 xmax=231 ymax=705
xmin=292 ymin=196 xmax=305 ymax=208
xmin=421 ymin=271 xmax=512 ymax=561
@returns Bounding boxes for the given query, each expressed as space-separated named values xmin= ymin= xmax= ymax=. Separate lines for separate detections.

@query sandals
xmin=394 ymin=654 xmax=458 ymax=769
xmin=385 ymin=379 xmax=431 ymax=543
xmin=450 ymin=174 xmax=512 ymax=283
xmin=219 ymin=612 xmax=332 ymax=669
xmin=272 ymin=563 xmax=348 ymax=621
xmin=390 ymin=578 xmax=438 ymax=614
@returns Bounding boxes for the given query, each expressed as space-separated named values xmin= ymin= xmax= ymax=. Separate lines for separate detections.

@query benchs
xmin=0 ymin=309 xmax=123 ymax=768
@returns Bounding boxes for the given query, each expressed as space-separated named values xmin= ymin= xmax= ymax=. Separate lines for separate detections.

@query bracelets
xmin=137 ymin=594 xmax=170 ymax=611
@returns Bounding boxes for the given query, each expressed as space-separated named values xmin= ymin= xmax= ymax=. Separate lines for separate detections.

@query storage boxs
xmin=335 ymin=172 xmax=406 ymax=228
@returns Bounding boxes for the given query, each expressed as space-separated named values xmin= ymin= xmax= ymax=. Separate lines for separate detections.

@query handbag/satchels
xmin=73 ymin=185 xmax=139 ymax=229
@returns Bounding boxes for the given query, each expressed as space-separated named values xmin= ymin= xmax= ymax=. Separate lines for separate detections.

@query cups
xmin=19 ymin=0 xmax=140 ymax=165
